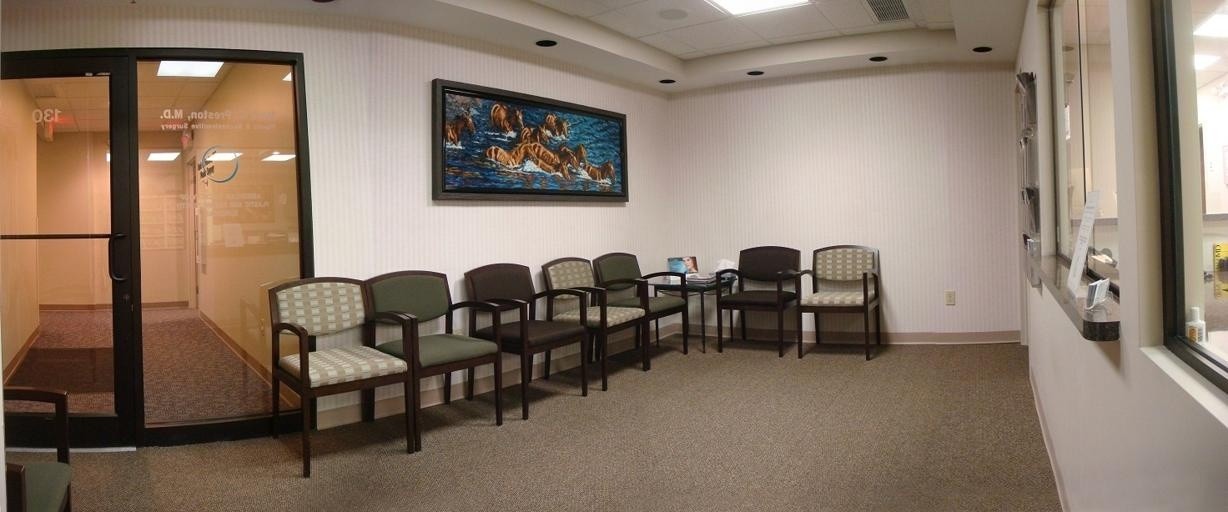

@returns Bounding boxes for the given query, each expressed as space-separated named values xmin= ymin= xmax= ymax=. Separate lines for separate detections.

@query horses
xmin=445 ymin=114 xmax=476 ymax=146
xmin=584 ymin=160 xmax=616 ymax=181
xmin=486 ymin=102 xmax=588 ymax=181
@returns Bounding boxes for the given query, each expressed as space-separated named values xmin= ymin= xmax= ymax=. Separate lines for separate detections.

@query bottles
xmin=1185 ymin=306 xmax=1206 ymax=342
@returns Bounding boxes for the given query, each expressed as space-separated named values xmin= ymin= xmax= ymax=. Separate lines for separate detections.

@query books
xmin=687 ymin=276 xmax=716 ymax=284
xmin=686 ymin=273 xmax=718 ymax=280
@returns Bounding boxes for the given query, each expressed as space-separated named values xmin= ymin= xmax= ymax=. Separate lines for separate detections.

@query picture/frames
xmin=429 ymin=78 xmax=629 ymax=204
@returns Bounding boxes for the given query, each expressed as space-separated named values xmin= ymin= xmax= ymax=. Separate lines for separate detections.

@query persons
xmin=681 ymin=256 xmax=699 ymax=273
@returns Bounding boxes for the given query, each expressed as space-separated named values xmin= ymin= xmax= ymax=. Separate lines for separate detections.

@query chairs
xmin=542 ymin=255 xmax=649 ymax=393
xmin=795 ymin=247 xmax=882 ymax=361
xmin=591 ymin=251 xmax=690 ymax=373
xmin=715 ymin=244 xmax=801 ymax=353
xmin=464 ymin=262 xmax=591 ymax=420
xmin=3 ymin=384 xmax=77 ymax=512
xmin=265 ymin=277 xmax=415 ymax=479
xmin=366 ymin=269 xmax=507 ymax=450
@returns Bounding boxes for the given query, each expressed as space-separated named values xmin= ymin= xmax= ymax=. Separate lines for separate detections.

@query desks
xmin=648 ymin=277 xmax=737 ymax=355
